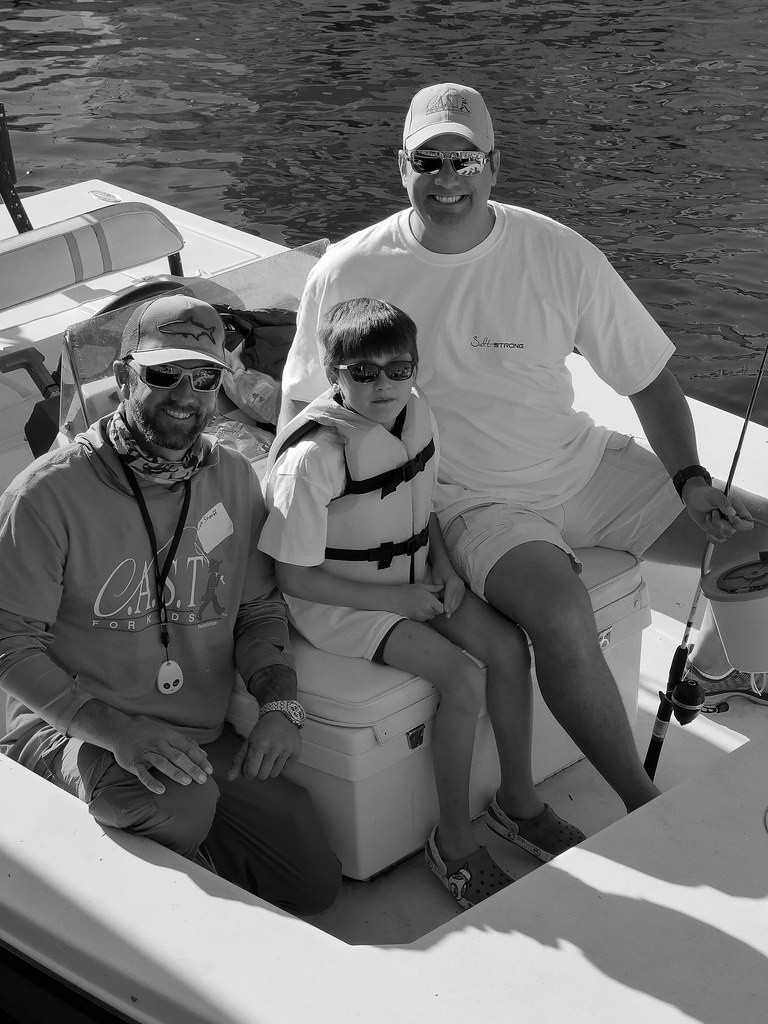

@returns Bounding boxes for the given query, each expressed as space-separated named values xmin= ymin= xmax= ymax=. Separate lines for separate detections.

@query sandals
xmin=481 ymin=789 xmax=587 ymax=863
xmin=424 ymin=823 xmax=514 ymax=909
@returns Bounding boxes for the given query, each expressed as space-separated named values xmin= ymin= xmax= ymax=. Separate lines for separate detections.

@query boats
xmin=2 ymin=175 xmax=768 ymax=1024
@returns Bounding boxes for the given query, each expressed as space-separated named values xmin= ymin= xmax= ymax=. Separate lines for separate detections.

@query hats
xmin=119 ymin=293 xmax=235 ymax=376
xmin=402 ymin=82 xmax=495 ymax=153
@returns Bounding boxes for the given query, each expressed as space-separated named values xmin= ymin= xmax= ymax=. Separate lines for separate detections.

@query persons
xmin=1 ymin=293 xmax=345 ymax=919
xmin=271 ymin=82 xmax=768 ymax=817
xmin=253 ymin=297 xmax=587 ymax=911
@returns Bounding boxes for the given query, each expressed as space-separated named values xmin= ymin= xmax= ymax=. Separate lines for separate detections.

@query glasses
xmin=405 ymin=148 xmax=493 ymax=178
xmin=334 ymin=360 xmax=416 ymax=385
xmin=124 ymin=359 xmax=224 ymax=394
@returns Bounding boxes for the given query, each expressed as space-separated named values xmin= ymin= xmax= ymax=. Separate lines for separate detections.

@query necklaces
xmin=118 ymin=451 xmax=192 ymax=695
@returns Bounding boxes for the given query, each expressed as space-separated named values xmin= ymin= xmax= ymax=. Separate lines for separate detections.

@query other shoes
xmin=685 ymin=658 xmax=768 ymax=709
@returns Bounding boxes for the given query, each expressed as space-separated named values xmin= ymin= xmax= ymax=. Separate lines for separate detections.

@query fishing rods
xmin=642 ymin=334 xmax=767 ymax=782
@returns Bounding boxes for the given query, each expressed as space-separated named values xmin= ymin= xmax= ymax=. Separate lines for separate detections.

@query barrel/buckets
xmin=699 ymin=516 xmax=767 ymax=674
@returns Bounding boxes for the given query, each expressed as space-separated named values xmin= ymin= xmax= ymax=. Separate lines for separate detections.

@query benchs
xmin=53 ymin=236 xmax=654 ymax=881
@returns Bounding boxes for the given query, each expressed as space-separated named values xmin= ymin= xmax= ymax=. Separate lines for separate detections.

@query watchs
xmin=673 ymin=466 xmax=712 ymax=506
xmin=258 ymin=697 xmax=305 ymax=728
